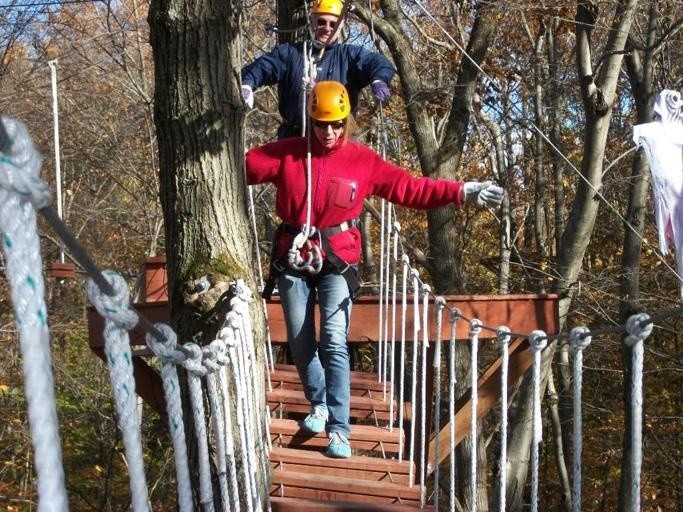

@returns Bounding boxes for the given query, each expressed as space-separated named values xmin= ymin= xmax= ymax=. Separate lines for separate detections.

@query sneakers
xmin=328 ymin=432 xmax=351 ymax=457
xmin=305 ymin=403 xmax=328 ymax=433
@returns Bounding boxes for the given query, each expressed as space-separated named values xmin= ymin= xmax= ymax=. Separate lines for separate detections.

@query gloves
xmin=460 ymin=181 xmax=504 ymax=209
xmin=372 ymin=80 xmax=390 ymax=102
xmin=242 ymin=85 xmax=253 ymax=109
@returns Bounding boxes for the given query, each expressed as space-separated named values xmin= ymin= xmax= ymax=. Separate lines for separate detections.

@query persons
xmin=245 ymin=80 xmax=504 ymax=460
xmin=238 ymin=0 xmax=398 ymax=145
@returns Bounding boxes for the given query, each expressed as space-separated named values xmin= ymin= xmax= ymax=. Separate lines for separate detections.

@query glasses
xmin=314 ymin=121 xmax=344 ymax=128
xmin=318 ymin=18 xmax=336 ymax=28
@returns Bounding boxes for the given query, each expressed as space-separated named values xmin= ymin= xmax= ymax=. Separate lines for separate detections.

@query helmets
xmin=312 ymin=0 xmax=343 ymax=16
xmin=308 ymin=81 xmax=351 ymax=122
